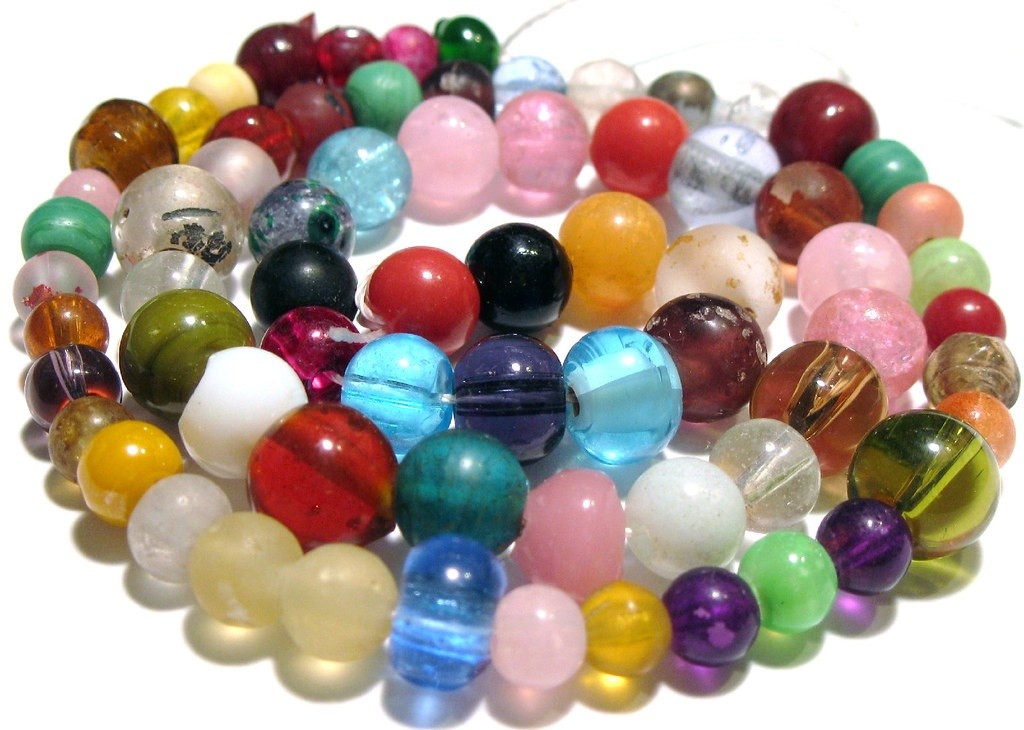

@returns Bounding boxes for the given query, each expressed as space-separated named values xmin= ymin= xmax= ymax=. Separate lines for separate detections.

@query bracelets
xmin=13 ymin=14 xmax=1020 ymax=691
